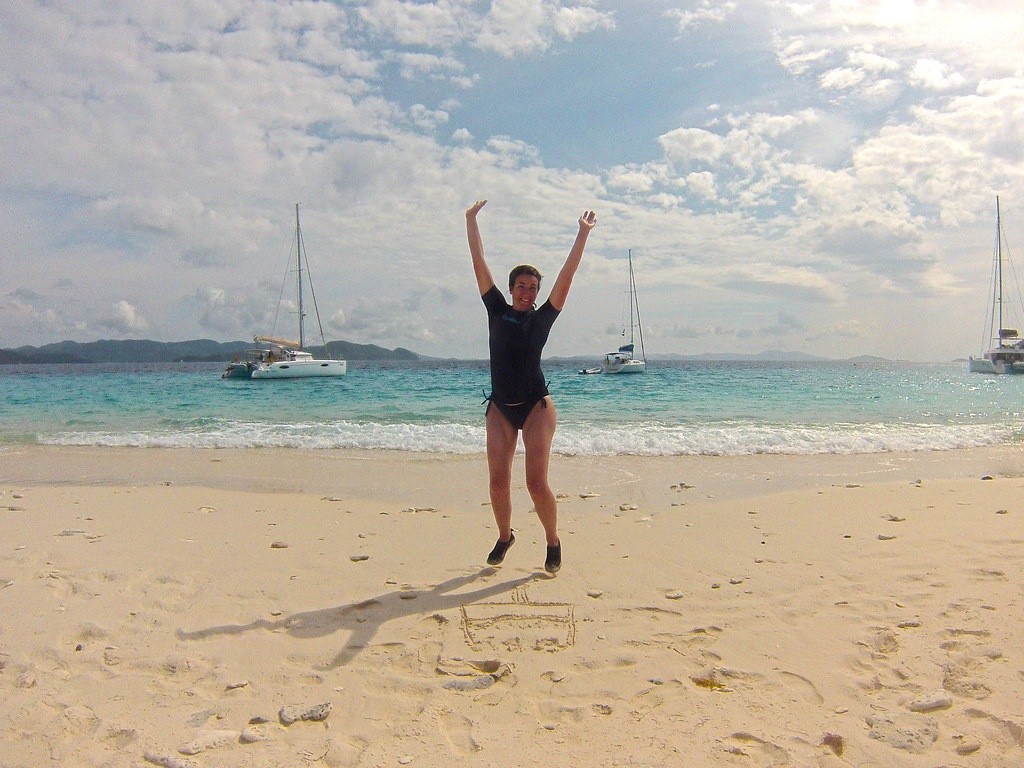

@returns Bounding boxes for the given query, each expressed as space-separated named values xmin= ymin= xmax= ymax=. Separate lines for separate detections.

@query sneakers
xmin=545 ymin=538 xmax=562 ymax=573
xmin=486 ymin=528 xmax=515 ymax=565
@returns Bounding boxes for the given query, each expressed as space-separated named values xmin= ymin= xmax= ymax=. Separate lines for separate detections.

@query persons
xmin=465 ymin=200 xmax=596 ymax=572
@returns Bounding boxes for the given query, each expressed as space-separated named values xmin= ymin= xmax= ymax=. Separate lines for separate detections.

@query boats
xmin=578 ymin=365 xmax=603 ymax=375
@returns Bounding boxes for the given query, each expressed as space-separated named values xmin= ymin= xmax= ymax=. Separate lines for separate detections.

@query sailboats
xmin=603 ymin=247 xmax=648 ymax=374
xmin=967 ymin=194 xmax=1024 ymax=374
xmin=223 ymin=200 xmax=346 ymax=379
